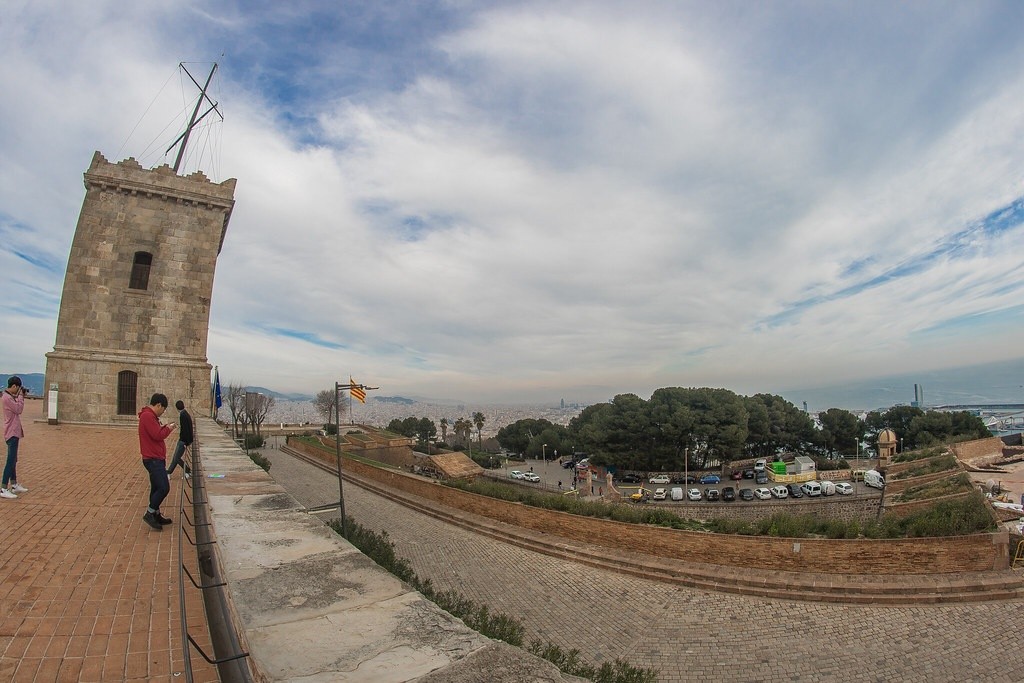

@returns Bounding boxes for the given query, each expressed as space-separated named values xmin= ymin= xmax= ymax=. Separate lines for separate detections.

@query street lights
xmin=335 ymin=382 xmax=379 ymax=540
xmin=685 ymin=448 xmax=689 ymax=497
xmin=855 ymin=437 xmax=859 ymax=498
xmin=543 ymin=444 xmax=547 ymax=490
xmin=232 ymin=391 xmax=263 ymax=455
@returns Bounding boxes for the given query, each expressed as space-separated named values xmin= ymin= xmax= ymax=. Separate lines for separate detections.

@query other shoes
xmin=181 ymin=472 xmax=192 ymax=479
xmin=167 ymin=474 xmax=171 ymax=480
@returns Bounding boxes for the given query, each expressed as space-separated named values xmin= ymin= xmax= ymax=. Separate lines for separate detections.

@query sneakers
xmin=0 ymin=488 xmax=18 ymax=498
xmin=154 ymin=512 xmax=171 ymax=525
xmin=143 ymin=510 xmax=163 ymax=530
xmin=12 ymin=484 xmax=28 ymax=493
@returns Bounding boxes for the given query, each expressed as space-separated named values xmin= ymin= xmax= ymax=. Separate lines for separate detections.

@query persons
xmin=558 ymin=480 xmax=562 ymax=487
xmin=1021 ymin=493 xmax=1024 ymax=510
xmin=530 ymin=467 xmax=533 ymax=471
xmin=599 ymin=486 xmax=603 ymax=495
xmin=166 ymin=400 xmax=194 ymax=473
xmin=138 ymin=393 xmax=180 ymax=531
xmin=736 ymin=480 xmax=740 ymax=489
xmin=0 ymin=375 xmax=28 ymax=498
xmin=641 ymin=489 xmax=645 ymax=494
xmin=559 ymin=459 xmax=562 ymax=465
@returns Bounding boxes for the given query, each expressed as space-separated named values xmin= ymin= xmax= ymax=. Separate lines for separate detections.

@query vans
xmin=851 ymin=469 xmax=866 ymax=482
xmin=799 ymin=481 xmax=854 ymax=497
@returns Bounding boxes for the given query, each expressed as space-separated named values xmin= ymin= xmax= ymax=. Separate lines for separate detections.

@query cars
xmin=687 ymin=459 xmax=803 ymax=501
xmin=649 ymin=474 xmax=671 ymax=484
xmin=561 ymin=458 xmax=590 ymax=469
xmin=670 ymin=487 xmax=683 ymax=501
xmin=619 ymin=474 xmax=642 ymax=483
xmin=654 ymin=488 xmax=667 ymax=500
xmin=674 ymin=476 xmax=695 ymax=484
xmin=510 ymin=471 xmax=540 ymax=483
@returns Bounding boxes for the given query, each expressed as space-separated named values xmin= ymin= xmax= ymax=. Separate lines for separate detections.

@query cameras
xmin=21 ymin=386 xmax=29 ymax=395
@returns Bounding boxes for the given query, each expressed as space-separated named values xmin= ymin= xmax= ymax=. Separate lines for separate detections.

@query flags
xmin=350 ymin=379 xmax=367 ymax=404
xmin=213 ymin=371 xmax=222 ymax=408
